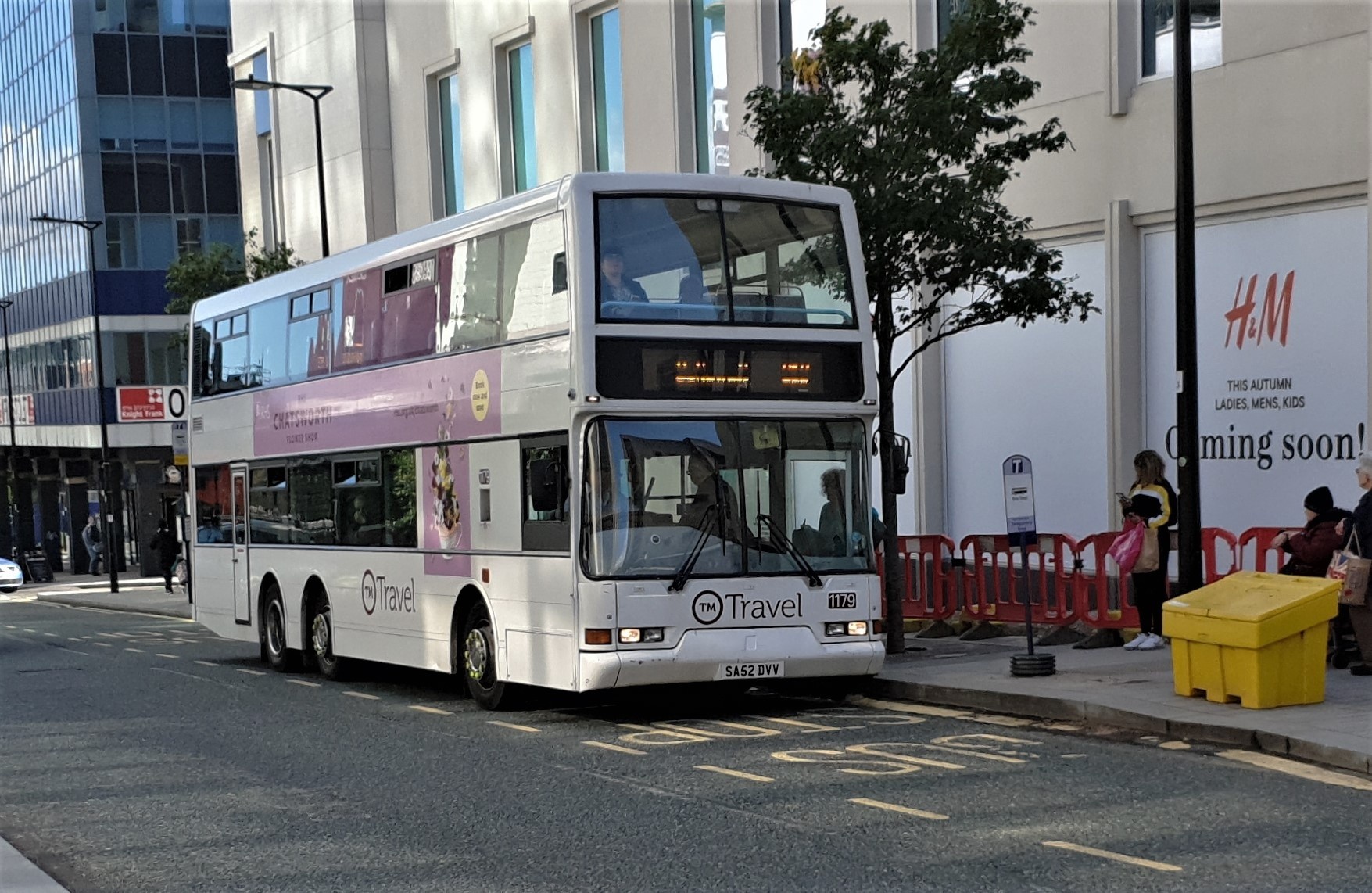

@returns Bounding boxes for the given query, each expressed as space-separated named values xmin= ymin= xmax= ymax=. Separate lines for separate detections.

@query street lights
xmin=30 ymin=214 xmax=121 ymax=593
xmin=231 ymin=75 xmax=332 ymax=259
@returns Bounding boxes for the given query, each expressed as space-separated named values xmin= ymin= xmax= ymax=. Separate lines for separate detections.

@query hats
xmin=1304 ymin=486 xmax=1334 ymax=515
xmin=157 ymin=518 xmax=167 ymax=528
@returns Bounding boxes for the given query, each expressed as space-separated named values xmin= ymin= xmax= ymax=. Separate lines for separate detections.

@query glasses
xmin=686 ymin=470 xmax=693 ymax=475
xmin=1355 ymin=468 xmax=1360 ymax=473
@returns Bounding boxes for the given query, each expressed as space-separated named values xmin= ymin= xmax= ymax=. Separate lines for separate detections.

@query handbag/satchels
xmin=1108 ymin=516 xmax=1143 ymax=574
xmin=1326 ymin=523 xmax=1371 ymax=606
xmin=92 ymin=541 xmax=104 ymax=552
xmin=1129 ymin=528 xmax=1159 ymax=574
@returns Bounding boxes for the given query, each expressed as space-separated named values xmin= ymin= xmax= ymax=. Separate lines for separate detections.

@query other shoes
xmin=1350 ymin=664 xmax=1372 ymax=675
xmin=1326 ymin=645 xmax=1344 ymax=664
xmin=91 ymin=571 xmax=102 ymax=576
xmin=164 ymin=589 xmax=173 ymax=594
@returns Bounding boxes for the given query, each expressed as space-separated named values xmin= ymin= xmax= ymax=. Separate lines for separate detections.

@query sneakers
xmin=1124 ymin=632 xmax=1167 ymax=650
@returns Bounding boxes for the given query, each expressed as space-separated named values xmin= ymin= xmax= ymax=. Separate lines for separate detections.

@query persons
xmin=1118 ymin=450 xmax=1177 ymax=650
xmin=208 ymin=517 xmax=223 ymax=542
xmin=1334 ymin=452 xmax=1372 ymax=676
xmin=82 ymin=516 xmax=102 ymax=576
xmin=678 ymin=452 xmax=739 ymax=542
xmin=150 ymin=518 xmax=179 ymax=595
xmin=1270 ymin=485 xmax=1344 ymax=581
xmin=819 ymin=469 xmax=886 ymax=555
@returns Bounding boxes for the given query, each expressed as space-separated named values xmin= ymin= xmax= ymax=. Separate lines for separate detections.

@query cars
xmin=0 ymin=559 xmax=25 ymax=594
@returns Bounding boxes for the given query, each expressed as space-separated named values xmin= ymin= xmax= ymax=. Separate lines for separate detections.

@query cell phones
xmin=1116 ymin=492 xmax=1131 ymax=508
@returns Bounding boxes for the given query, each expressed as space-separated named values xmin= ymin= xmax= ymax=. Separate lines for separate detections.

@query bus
xmin=189 ymin=170 xmax=911 ymax=705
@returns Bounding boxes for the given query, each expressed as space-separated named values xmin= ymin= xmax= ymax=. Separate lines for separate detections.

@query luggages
xmin=175 ymin=555 xmax=188 ymax=585
